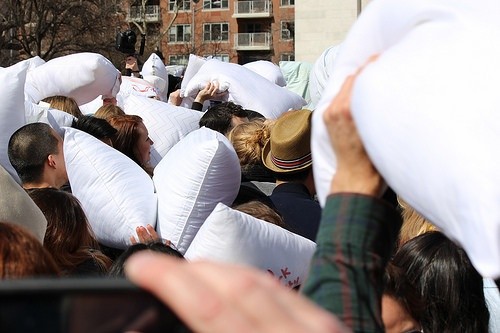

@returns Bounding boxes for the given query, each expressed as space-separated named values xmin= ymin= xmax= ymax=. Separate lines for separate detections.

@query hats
xmin=262 ymin=109 xmax=312 ymax=172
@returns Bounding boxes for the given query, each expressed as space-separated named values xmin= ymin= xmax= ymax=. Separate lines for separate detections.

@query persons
xmin=0 ymin=53 xmax=500 ymax=333
